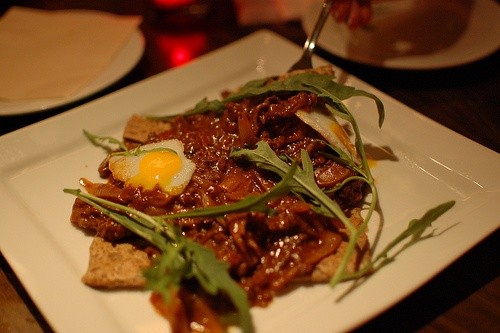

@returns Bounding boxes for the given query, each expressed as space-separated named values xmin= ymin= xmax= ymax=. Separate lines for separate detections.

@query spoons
xmin=287 ymin=0 xmax=336 ymax=73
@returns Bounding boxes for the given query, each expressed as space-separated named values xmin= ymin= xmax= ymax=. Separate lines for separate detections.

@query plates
xmin=0 ymin=10 xmax=146 ymax=115
xmin=301 ymin=0 xmax=500 ymax=70
xmin=0 ymin=29 xmax=499 ymax=333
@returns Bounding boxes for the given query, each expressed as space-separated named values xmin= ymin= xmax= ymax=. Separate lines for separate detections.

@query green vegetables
xmin=63 ymin=62 xmax=457 ymax=333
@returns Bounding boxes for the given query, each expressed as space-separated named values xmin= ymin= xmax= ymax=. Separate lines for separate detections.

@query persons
xmin=330 ymin=0 xmax=375 ymax=30
